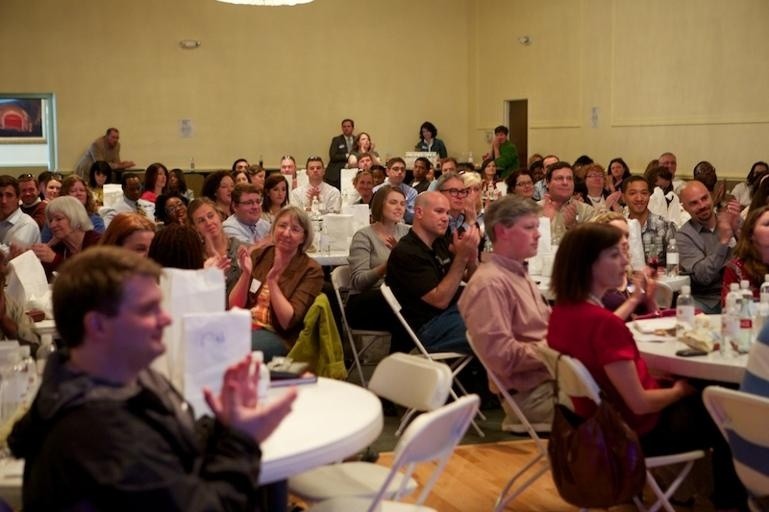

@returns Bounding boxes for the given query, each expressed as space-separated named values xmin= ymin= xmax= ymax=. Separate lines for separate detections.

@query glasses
xmin=238 ymin=200 xmax=261 ymax=205
xmin=441 ymin=188 xmax=469 ymax=198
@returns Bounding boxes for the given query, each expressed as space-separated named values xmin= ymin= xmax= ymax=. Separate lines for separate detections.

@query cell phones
xmin=676 ymin=349 xmax=707 ymax=356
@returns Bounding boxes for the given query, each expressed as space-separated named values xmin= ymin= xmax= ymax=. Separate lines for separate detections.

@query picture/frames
xmin=0 ymin=98 xmax=47 ymax=146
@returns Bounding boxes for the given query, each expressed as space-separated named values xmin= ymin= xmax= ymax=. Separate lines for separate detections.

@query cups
xmin=0 ymin=340 xmax=20 ymax=362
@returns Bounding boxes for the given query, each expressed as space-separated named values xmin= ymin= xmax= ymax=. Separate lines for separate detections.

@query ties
xmin=135 ymin=202 xmax=145 ymax=215
xmin=250 ymin=224 xmax=261 ymax=242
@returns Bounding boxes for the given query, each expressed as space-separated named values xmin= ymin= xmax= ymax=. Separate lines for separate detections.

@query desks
xmin=461 ymin=272 xmax=691 ymax=303
xmin=305 ymin=233 xmax=352 ymax=265
xmin=0 ymin=376 xmax=383 ymax=512
xmin=113 ymin=170 xmax=279 ymax=199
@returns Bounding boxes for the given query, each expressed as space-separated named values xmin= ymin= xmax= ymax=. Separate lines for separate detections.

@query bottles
xmin=719 ymin=274 xmax=769 ymax=358
xmin=320 ymin=224 xmax=330 ymax=256
xmin=251 ymin=351 xmax=270 ymax=397
xmin=676 ymin=285 xmax=694 ymax=352
xmin=15 ymin=334 xmax=55 ymax=403
xmin=666 ymin=239 xmax=678 ymax=281
xmin=306 ymin=195 xmax=319 ymax=212
xmin=468 ymin=153 xmax=473 ymax=162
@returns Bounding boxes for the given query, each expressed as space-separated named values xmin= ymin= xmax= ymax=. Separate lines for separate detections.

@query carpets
xmin=346 ymin=360 xmax=546 ymax=455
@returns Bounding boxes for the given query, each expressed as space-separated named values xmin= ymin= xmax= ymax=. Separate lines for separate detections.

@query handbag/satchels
xmin=548 ymin=399 xmax=645 ymax=507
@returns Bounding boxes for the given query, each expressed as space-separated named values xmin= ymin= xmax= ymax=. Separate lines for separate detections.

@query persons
xmin=456 ymin=194 xmax=560 ymax=424
xmin=482 ymin=125 xmax=520 ymax=179
xmin=546 ymin=222 xmax=748 ymax=512
xmin=18 ymin=246 xmax=298 ymax=511
xmin=73 ymin=128 xmax=136 ymax=178
xmin=385 ymin=191 xmax=480 ymax=357
xmin=416 ymin=121 xmax=448 ymax=159
xmin=730 ymin=317 xmax=769 ymax=498
xmin=1 ymin=157 xmax=327 ymax=363
xmin=329 ymin=152 xmax=769 ymax=319
xmin=348 ymin=132 xmax=381 ymax=169
xmin=323 ymin=119 xmax=356 ymax=192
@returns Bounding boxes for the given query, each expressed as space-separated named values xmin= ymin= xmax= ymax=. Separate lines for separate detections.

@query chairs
xmin=288 ymin=352 xmax=453 ymax=507
xmin=331 ymin=265 xmax=391 ymax=388
xmin=700 ymin=385 xmax=769 ymax=445
xmin=466 ymin=329 xmax=553 ymax=512
xmin=381 ymin=282 xmax=487 ymax=439
xmin=303 ymin=393 xmax=481 ymax=512
xmin=656 ymin=281 xmax=675 ymax=311
xmin=531 ymin=342 xmax=703 ymax=512
